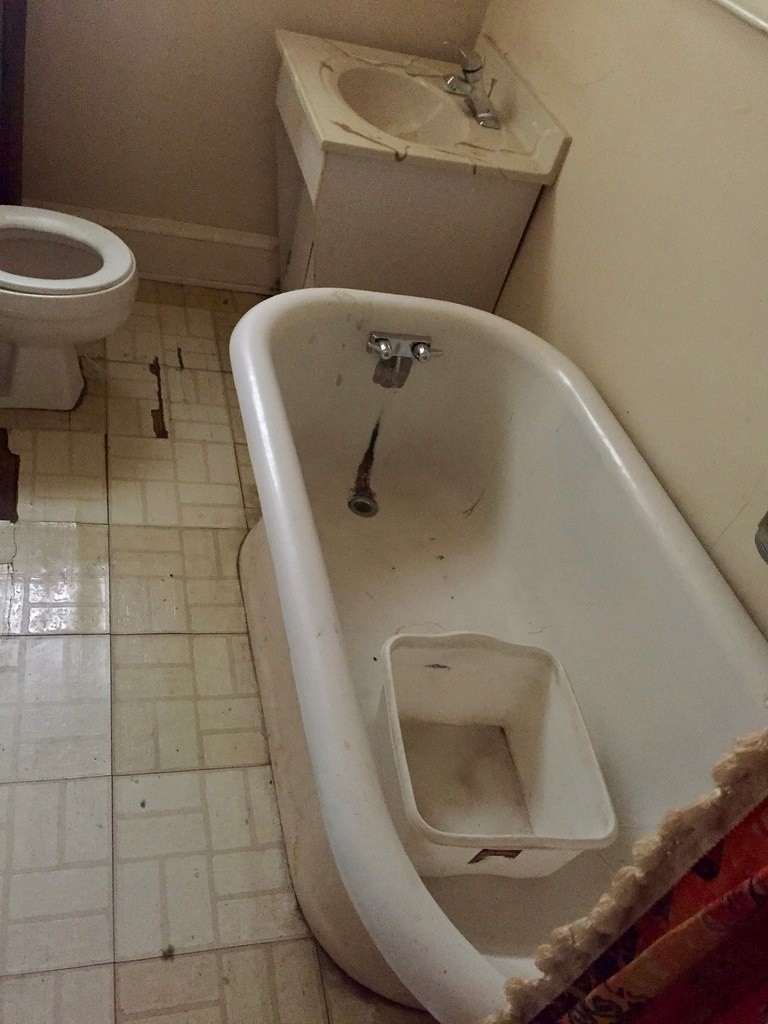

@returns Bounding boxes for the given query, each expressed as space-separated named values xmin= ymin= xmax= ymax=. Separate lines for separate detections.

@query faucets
xmin=443 ymin=47 xmax=500 ymax=129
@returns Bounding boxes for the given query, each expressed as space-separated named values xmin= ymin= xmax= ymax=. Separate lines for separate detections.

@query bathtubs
xmin=232 ymin=289 xmax=768 ymax=1023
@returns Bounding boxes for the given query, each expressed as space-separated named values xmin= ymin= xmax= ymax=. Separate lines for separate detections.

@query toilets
xmin=1 ymin=206 xmax=135 ymax=408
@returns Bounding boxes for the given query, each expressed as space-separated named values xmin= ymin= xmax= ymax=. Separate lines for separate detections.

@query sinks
xmin=323 ymin=58 xmax=478 ymax=145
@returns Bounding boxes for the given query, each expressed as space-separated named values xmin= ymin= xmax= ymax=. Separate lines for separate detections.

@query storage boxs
xmin=367 ymin=629 xmax=618 ymax=879
